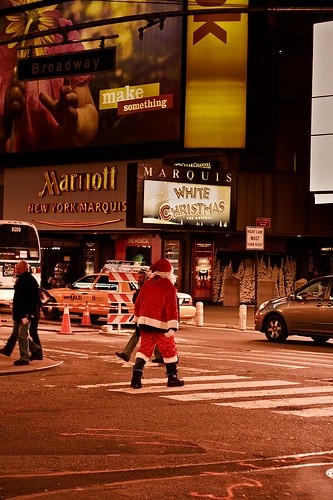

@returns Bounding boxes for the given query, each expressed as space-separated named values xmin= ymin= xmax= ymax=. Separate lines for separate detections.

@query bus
xmin=0 ymin=219 xmax=41 ymax=307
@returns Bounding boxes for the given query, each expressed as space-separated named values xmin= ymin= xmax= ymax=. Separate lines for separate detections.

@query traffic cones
xmin=79 ymin=306 xmax=94 ymax=328
xmin=56 ymin=304 xmax=73 ymax=335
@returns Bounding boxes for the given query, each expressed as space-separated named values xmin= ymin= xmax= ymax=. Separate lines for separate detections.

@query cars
xmin=40 ymin=272 xmax=196 ymax=322
xmin=254 ymin=274 xmax=333 ymax=344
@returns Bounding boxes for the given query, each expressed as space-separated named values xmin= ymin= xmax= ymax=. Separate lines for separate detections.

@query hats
xmin=149 ymin=259 xmax=174 ymax=276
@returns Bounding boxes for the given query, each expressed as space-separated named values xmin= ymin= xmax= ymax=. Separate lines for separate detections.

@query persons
xmin=1 ymin=261 xmax=46 ymax=366
xmin=0 ymin=0 xmax=100 ymax=154
xmin=116 ymin=259 xmax=188 ymax=388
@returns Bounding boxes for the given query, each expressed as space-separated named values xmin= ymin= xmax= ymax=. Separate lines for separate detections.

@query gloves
xmin=164 ymin=329 xmax=175 ymax=337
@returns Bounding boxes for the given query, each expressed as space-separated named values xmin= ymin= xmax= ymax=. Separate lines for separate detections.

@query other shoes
xmin=29 ymin=353 xmax=43 ymax=361
xmin=152 ymin=358 xmax=164 ymax=363
xmin=115 ymin=352 xmax=130 ymax=362
xmin=0 ymin=349 xmax=12 ymax=357
xmin=13 ymin=359 xmax=30 ymax=366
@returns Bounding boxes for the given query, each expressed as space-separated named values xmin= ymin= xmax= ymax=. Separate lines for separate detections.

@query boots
xmin=167 ymin=374 xmax=185 ymax=387
xmin=131 ymin=370 xmax=143 ymax=388
